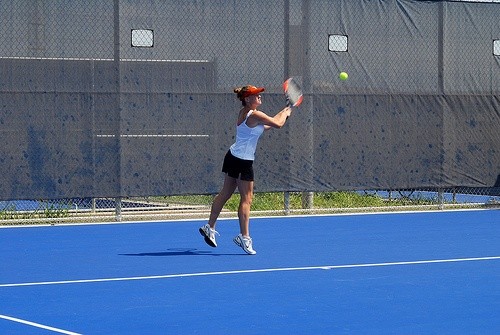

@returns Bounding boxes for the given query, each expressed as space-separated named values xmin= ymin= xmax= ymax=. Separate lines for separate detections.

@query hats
xmin=240 ymin=85 xmax=264 ymax=100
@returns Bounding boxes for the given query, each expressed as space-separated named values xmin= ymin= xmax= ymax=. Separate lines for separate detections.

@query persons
xmin=199 ymin=85 xmax=292 ymax=255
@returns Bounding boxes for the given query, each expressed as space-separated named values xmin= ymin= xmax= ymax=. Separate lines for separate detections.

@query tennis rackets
xmin=282 ymin=77 xmax=305 ymax=120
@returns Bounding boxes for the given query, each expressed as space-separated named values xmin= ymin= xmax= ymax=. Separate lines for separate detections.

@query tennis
xmin=339 ymin=71 xmax=348 ymax=80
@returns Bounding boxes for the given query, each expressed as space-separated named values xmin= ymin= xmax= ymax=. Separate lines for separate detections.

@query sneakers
xmin=233 ymin=233 xmax=256 ymax=254
xmin=199 ymin=224 xmax=220 ymax=247
